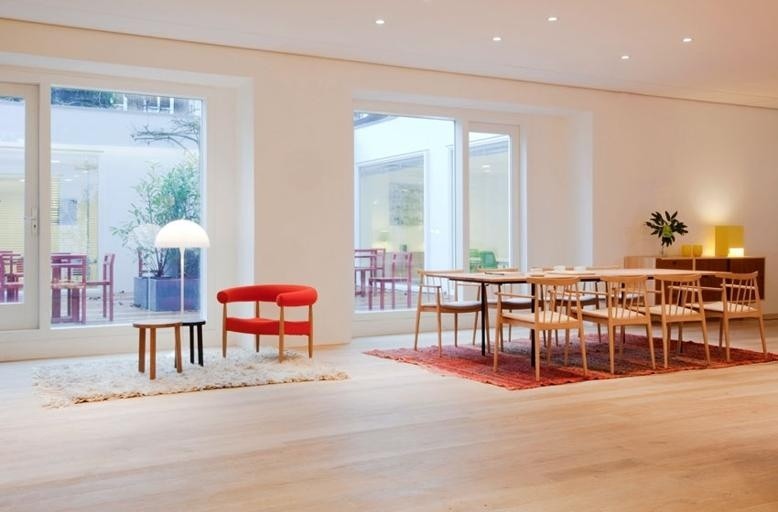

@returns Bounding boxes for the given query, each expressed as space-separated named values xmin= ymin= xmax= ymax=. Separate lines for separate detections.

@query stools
xmin=134 ymin=320 xmax=184 ymax=379
xmin=174 ymin=320 xmax=206 ymax=369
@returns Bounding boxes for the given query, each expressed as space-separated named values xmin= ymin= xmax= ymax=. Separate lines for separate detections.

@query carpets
xmin=363 ymin=331 xmax=778 ymax=389
xmin=33 ymin=341 xmax=348 ymax=411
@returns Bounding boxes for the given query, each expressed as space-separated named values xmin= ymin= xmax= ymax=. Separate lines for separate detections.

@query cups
xmin=554 ymin=265 xmax=566 ymax=272
xmin=573 ymin=266 xmax=587 ymax=272
xmin=692 ymin=245 xmax=702 ymax=257
xmin=530 ymin=268 xmax=543 ymax=274
xmin=681 ymin=244 xmax=691 ymax=256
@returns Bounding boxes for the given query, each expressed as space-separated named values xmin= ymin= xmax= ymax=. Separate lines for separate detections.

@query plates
xmin=530 ymin=274 xmax=545 ymax=277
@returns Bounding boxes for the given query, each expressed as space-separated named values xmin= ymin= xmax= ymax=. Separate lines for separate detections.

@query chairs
xmin=415 ymin=270 xmax=492 ymax=357
xmin=368 ymin=252 xmax=412 ymax=310
xmin=355 ymin=249 xmax=385 ymax=296
xmin=217 ymin=285 xmax=317 ymax=363
xmin=606 ymin=280 xmax=646 ymax=342
xmin=1 ymin=251 xmax=87 ymax=326
xmin=623 ymin=272 xmax=711 ymax=368
xmin=543 ymin=268 xmax=602 ymax=346
xmin=493 ymin=275 xmax=589 ymax=382
xmin=676 ymin=271 xmax=768 ymax=363
xmin=472 ymin=267 xmax=546 ymax=352
xmin=76 ymin=255 xmax=115 ymax=327
xmin=563 ymin=277 xmax=656 ymax=375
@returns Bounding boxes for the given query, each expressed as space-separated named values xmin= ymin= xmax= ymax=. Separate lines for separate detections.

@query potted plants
xmin=645 ymin=211 xmax=688 ymax=259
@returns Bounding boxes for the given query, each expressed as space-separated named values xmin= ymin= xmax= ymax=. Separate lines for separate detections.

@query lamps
xmin=152 ymin=220 xmax=212 ymax=318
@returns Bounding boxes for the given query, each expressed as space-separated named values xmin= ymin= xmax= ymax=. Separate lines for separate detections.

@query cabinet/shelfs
xmin=656 ymin=256 xmax=765 ymax=303
